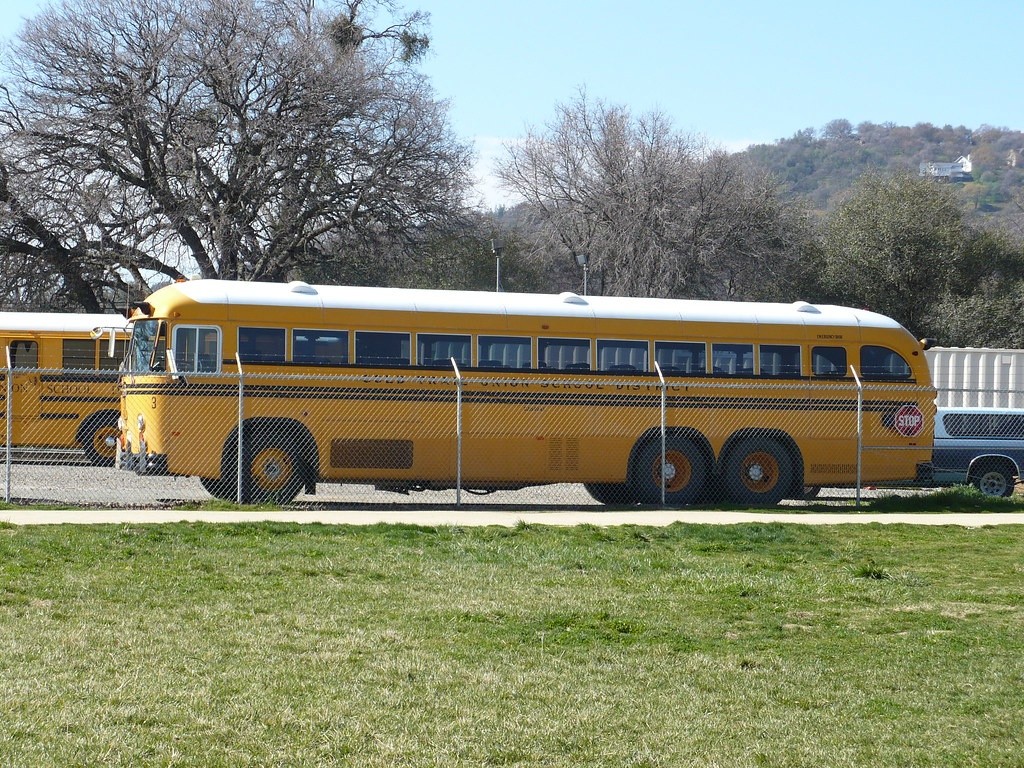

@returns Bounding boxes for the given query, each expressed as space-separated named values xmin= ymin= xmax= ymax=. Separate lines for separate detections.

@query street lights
xmin=576 ymin=254 xmax=589 ymax=296
xmin=490 ymin=237 xmax=503 ymax=293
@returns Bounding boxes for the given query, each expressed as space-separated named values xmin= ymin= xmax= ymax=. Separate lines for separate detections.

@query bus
xmin=0 ymin=312 xmax=343 ymax=465
xmin=90 ymin=279 xmax=938 ymax=508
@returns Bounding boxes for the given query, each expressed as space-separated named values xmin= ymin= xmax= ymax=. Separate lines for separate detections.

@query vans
xmin=786 ymin=405 xmax=1024 ymax=500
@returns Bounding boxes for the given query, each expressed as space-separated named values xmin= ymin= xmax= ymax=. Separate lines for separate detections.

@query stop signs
xmin=895 ymin=404 xmax=925 ymax=438
